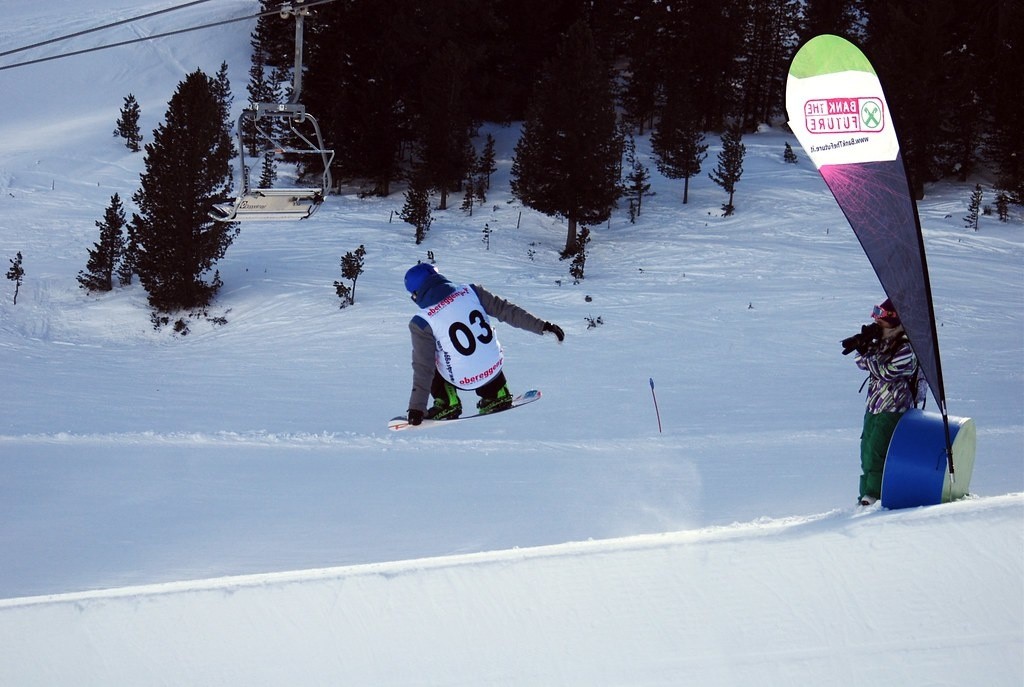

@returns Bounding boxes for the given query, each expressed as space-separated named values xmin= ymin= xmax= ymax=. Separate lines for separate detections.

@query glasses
xmin=411 ymin=292 xmax=418 ymax=302
xmin=870 ymin=305 xmax=898 ymax=318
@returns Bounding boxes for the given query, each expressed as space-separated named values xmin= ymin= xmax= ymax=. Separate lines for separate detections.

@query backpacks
xmin=870 ymin=338 xmax=928 ymax=405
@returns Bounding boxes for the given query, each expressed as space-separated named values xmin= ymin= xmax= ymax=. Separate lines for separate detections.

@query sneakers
xmin=424 ymin=391 xmax=462 ymax=420
xmin=477 ymin=384 xmax=513 ymax=414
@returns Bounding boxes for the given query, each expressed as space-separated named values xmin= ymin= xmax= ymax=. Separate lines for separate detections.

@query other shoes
xmin=861 ymin=495 xmax=876 ymax=505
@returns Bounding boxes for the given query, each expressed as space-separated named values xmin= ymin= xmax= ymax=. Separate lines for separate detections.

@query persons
xmin=855 ymin=295 xmax=919 ymax=507
xmin=403 ymin=261 xmax=564 ymax=426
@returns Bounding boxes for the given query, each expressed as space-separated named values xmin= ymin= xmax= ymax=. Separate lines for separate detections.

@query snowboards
xmin=388 ymin=389 xmax=543 ymax=433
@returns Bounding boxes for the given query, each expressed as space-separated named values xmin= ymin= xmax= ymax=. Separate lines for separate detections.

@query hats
xmin=405 ymin=263 xmax=438 ymax=293
xmin=878 ymin=298 xmax=901 ymax=325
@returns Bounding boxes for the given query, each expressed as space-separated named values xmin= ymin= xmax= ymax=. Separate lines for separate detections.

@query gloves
xmin=861 ymin=323 xmax=876 ymax=341
xmin=407 ymin=409 xmax=424 ymax=426
xmin=542 ymin=321 xmax=565 ymax=344
xmin=854 ymin=334 xmax=868 ymax=356
